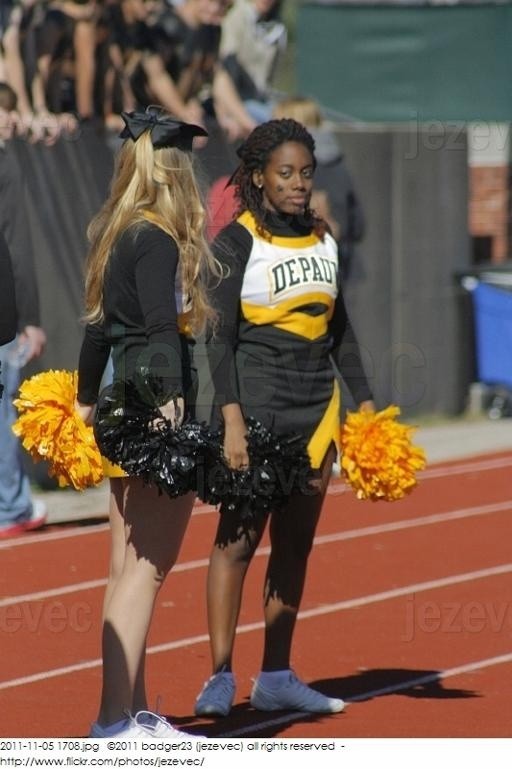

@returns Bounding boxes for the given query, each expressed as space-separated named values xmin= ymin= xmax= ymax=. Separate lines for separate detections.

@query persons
xmin=0 ymin=149 xmax=50 ymax=543
xmin=270 ymin=103 xmax=365 ymax=298
xmin=72 ymin=108 xmax=214 ymax=739
xmin=0 ymin=1 xmax=292 ymax=150
xmin=191 ymin=117 xmax=375 ymax=721
xmin=200 ymin=143 xmax=242 ymax=438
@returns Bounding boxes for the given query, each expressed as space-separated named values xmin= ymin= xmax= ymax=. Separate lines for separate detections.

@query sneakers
xmin=88 ymin=717 xmax=157 ymax=737
xmin=250 ymin=670 xmax=345 ymax=714
xmin=193 ymin=672 xmax=235 ymax=718
xmin=0 ymin=496 xmax=49 ymax=538
xmin=134 ymin=710 xmax=207 ymax=739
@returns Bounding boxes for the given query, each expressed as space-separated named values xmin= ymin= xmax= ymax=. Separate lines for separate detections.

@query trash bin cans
xmin=456 ymin=265 xmax=512 ymax=420
